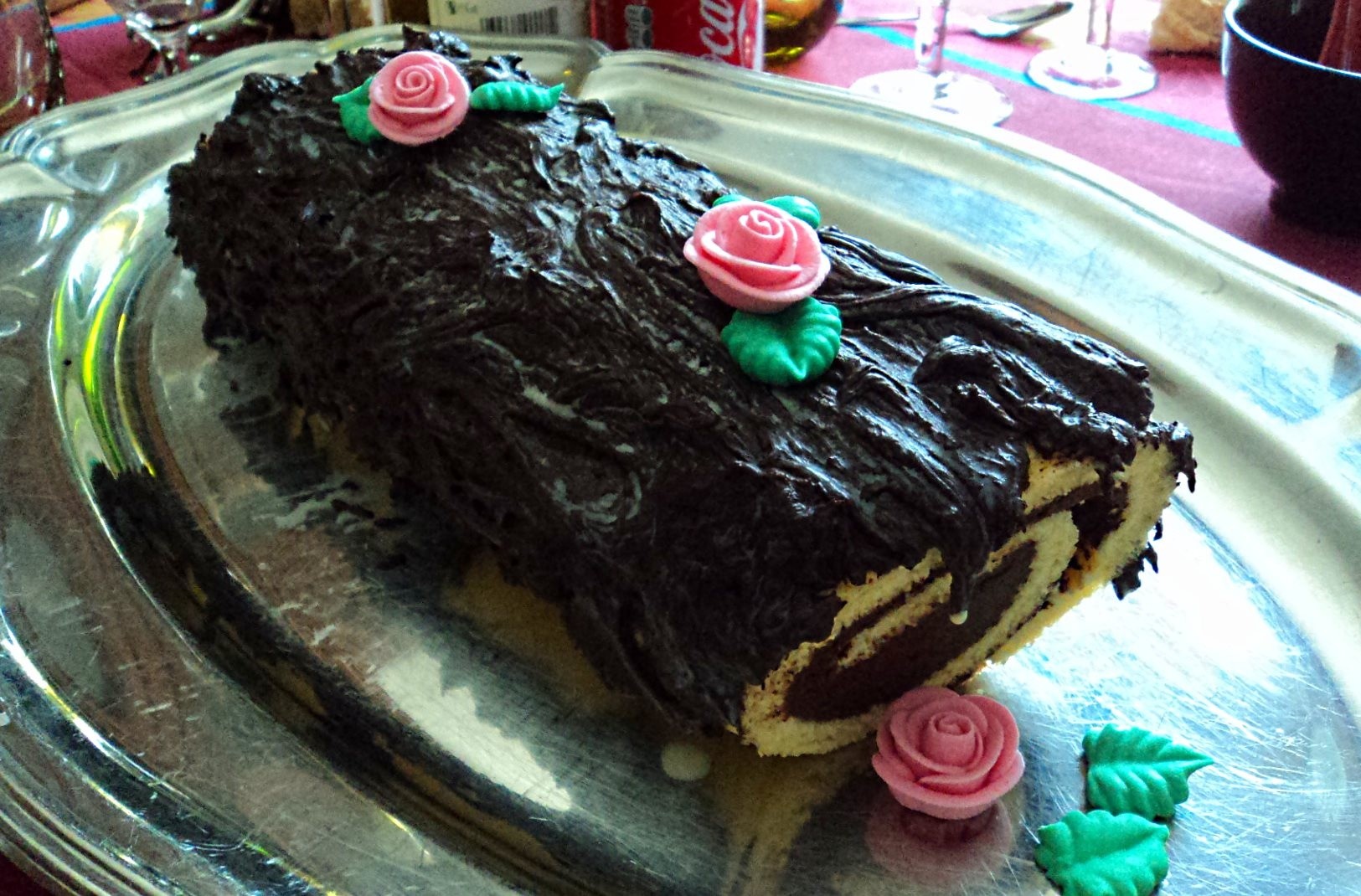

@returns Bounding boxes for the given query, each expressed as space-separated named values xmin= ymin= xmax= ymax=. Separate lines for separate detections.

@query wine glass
xmin=102 ymin=0 xmax=213 ymax=84
xmin=1025 ymin=0 xmax=1157 ymax=99
xmin=849 ymin=1 xmax=1011 ymax=124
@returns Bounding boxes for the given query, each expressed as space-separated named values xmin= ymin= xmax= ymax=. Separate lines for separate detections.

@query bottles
xmin=763 ymin=1 xmax=839 ymax=63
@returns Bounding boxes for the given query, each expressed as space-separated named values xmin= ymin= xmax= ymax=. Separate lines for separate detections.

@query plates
xmin=1 ymin=19 xmax=1360 ymax=896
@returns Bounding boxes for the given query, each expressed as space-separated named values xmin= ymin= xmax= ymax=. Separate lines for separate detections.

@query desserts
xmin=165 ymin=20 xmax=1215 ymax=896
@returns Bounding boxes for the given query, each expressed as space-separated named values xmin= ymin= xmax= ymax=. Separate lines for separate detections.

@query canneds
xmin=590 ymin=0 xmax=764 ymax=72
xmin=427 ymin=0 xmax=588 ymax=35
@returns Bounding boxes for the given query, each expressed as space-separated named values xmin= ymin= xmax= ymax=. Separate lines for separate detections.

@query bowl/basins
xmin=1221 ymin=1 xmax=1361 ymax=231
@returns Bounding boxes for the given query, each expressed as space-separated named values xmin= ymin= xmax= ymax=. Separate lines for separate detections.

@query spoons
xmin=832 ymin=1 xmax=1073 ymax=40
